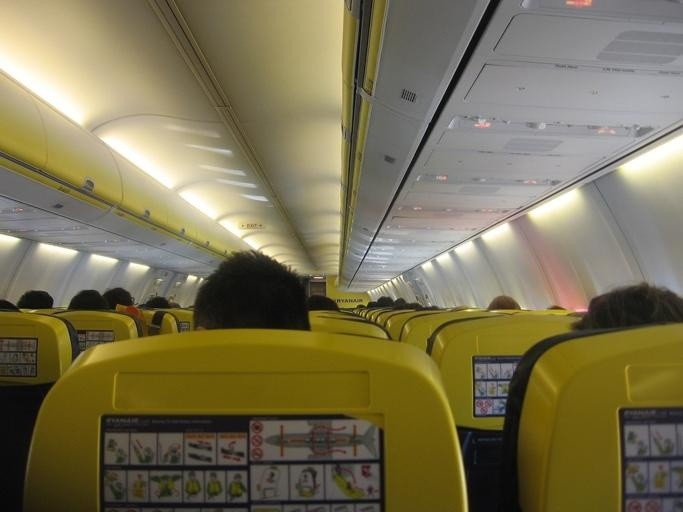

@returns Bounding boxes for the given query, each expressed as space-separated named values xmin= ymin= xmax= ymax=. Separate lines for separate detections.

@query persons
xmin=0 ymin=285 xmax=182 ymax=323
xmin=566 ymin=279 xmax=683 ymax=338
xmin=191 ymin=249 xmax=310 ymax=331
xmin=307 ymin=294 xmax=340 ymax=312
xmin=486 ymin=294 xmax=521 ymax=311
xmin=372 ymin=295 xmax=438 ymax=311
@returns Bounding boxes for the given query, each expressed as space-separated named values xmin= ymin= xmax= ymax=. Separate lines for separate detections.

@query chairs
xmin=0 ymin=307 xmax=683 ymax=512
